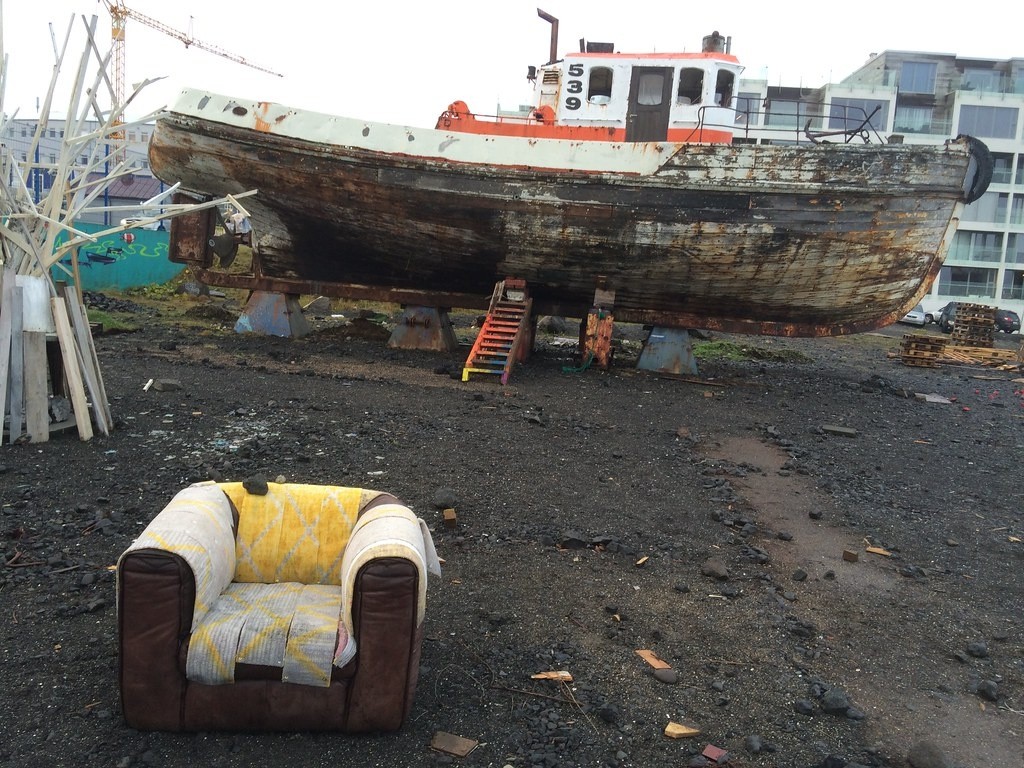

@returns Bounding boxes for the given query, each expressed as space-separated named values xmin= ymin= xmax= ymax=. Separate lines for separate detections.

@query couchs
xmin=114 ymin=479 xmax=441 ymax=736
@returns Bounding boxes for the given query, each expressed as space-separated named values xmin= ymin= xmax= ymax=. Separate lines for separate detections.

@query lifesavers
xmin=957 ymin=133 xmax=994 ymax=202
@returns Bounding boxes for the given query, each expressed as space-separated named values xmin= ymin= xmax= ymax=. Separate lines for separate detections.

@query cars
xmin=925 ymin=306 xmax=946 ymax=325
xmin=896 ymin=303 xmax=926 ymax=326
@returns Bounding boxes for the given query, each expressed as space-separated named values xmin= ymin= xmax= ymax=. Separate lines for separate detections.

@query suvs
xmin=983 ymin=308 xmax=1021 ymax=334
xmin=938 ymin=301 xmax=971 ymax=333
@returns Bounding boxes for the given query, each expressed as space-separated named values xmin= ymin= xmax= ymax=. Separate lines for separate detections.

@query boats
xmin=147 ymin=6 xmax=997 ymax=340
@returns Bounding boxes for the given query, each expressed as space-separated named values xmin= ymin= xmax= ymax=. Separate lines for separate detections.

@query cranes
xmin=99 ymin=0 xmax=285 ymax=170
